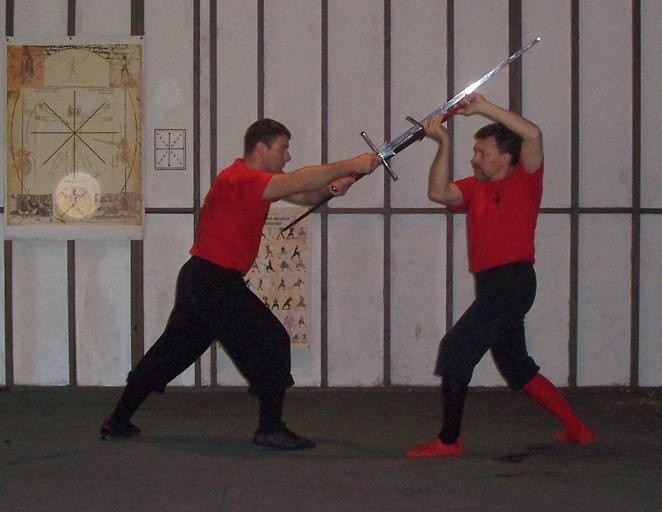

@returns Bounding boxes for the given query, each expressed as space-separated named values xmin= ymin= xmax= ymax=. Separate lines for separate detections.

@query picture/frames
xmin=4 ymin=35 xmax=145 ymax=240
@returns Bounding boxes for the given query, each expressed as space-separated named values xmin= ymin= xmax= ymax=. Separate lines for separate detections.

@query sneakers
xmin=402 ymin=432 xmax=465 ymax=458
xmin=552 ymin=428 xmax=595 ymax=446
xmin=99 ymin=419 xmax=142 ymax=439
xmin=252 ymin=426 xmax=315 ymax=451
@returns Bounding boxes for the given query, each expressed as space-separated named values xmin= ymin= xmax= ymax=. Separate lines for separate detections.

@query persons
xmin=401 ymin=91 xmax=602 ymax=460
xmin=99 ymin=119 xmax=382 ymax=450
